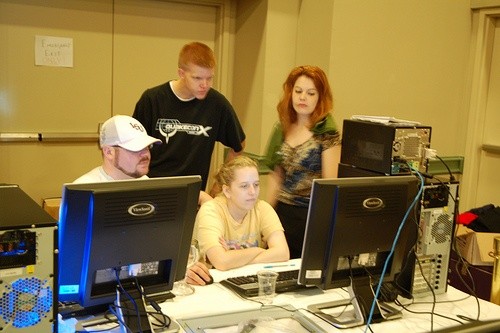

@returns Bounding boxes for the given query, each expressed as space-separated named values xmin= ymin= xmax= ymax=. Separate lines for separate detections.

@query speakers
xmin=342 ymin=119 xmax=431 ymax=174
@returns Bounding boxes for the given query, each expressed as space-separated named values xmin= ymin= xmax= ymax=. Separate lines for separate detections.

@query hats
xmin=99 ymin=114 xmax=162 ymax=151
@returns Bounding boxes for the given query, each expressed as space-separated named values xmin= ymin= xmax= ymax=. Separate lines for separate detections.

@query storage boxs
xmin=454 ymin=222 xmax=500 ymax=265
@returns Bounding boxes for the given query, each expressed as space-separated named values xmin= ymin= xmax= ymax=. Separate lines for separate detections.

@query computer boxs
xmin=0 ymin=183 xmax=59 ymax=333
xmin=339 ymin=164 xmax=460 ymax=297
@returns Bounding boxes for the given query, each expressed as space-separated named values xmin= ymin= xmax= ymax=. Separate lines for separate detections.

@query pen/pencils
xmin=264 ymin=264 xmax=296 ymax=268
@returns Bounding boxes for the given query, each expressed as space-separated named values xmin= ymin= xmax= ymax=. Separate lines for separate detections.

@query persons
xmin=129 ymin=41 xmax=246 ymax=198
xmin=70 ymin=114 xmax=216 ymax=286
xmin=188 ymin=156 xmax=290 ymax=272
xmin=256 ymin=65 xmax=342 ymax=260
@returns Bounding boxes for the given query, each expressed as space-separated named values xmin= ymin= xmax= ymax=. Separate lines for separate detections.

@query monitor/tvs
xmin=301 ymin=176 xmax=418 ymax=282
xmin=57 ymin=176 xmax=203 ymax=291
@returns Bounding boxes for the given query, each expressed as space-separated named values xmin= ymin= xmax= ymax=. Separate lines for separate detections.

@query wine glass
xmin=170 ymin=238 xmax=201 ymax=296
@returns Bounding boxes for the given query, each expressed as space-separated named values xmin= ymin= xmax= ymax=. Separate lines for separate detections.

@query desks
xmin=56 ymin=256 xmax=500 ymax=333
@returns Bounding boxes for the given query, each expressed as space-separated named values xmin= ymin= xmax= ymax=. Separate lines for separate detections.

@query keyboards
xmin=57 ymin=294 xmax=176 ymax=317
xmin=221 ymin=269 xmax=319 ymax=297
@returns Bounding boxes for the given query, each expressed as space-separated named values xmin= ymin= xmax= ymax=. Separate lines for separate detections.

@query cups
xmin=256 ymin=270 xmax=279 ymax=303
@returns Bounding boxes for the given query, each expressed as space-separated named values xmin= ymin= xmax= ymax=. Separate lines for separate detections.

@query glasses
xmin=112 ymin=143 xmax=154 ymax=149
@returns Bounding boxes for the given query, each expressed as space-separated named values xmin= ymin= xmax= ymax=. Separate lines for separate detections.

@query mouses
xmin=196 ymin=274 xmax=214 ymax=285
